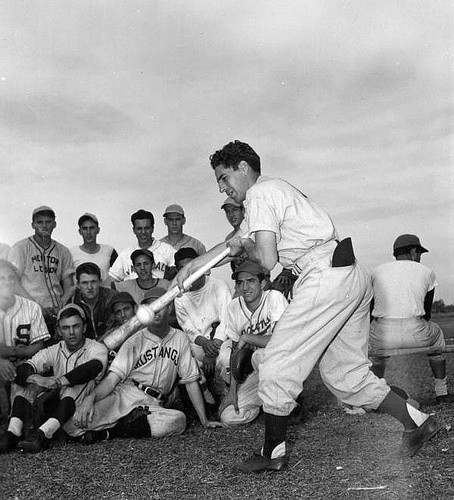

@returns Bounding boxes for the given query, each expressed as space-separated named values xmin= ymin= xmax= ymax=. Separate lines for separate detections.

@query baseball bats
xmin=103 ymin=231 xmax=252 ymax=351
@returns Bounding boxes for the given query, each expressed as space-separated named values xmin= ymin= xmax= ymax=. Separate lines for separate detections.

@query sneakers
xmin=397 ymin=415 xmax=441 ymax=458
xmin=17 ymin=429 xmax=53 ymax=454
xmin=231 ymin=452 xmax=290 ymax=472
xmin=0 ymin=431 xmax=23 ymax=454
xmin=84 ymin=430 xmax=107 ymax=446
xmin=436 ymin=391 xmax=454 ymax=402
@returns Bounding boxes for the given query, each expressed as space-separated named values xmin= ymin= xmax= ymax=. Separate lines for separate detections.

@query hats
xmin=221 ymin=197 xmax=243 ymax=209
xmin=163 ymin=204 xmax=184 ymax=217
xmin=56 ymin=303 xmax=86 ymax=326
xmin=0 ymin=259 xmax=17 ymax=271
xmin=33 ymin=206 xmax=53 ymax=220
xmin=141 ymin=288 xmax=170 ymax=304
xmin=174 ymin=247 xmax=199 ymax=261
xmin=109 ymin=292 xmax=135 ymax=313
xmin=232 ymin=260 xmax=262 ymax=280
xmin=130 ymin=249 xmax=154 ymax=263
xmin=78 ymin=213 xmax=98 ymax=227
xmin=393 ymin=234 xmax=429 ymax=255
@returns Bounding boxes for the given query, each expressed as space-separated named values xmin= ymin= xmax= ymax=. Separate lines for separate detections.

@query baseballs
xmin=136 ymin=304 xmax=155 ymax=325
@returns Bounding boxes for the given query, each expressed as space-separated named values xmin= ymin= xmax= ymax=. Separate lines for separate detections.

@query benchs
xmin=368 ymin=345 xmax=454 ymax=358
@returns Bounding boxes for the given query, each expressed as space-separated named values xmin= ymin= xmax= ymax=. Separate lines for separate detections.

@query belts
xmin=132 ymin=380 xmax=166 ymax=400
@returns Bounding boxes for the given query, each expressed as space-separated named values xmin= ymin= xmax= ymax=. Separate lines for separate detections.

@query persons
xmin=71 ymin=213 xmax=124 ymax=290
xmin=217 ymin=261 xmax=303 ymax=427
xmin=157 ymin=204 xmax=211 ymax=275
xmin=270 ymin=265 xmax=299 ymax=304
xmin=221 ymin=197 xmax=257 ymax=271
xmin=0 ymin=303 xmax=108 ymax=455
xmin=49 ymin=287 xmax=229 ymax=452
xmin=174 ymin=248 xmax=232 ymax=386
xmin=98 ymin=292 xmax=216 ymax=406
xmin=103 ymin=209 xmax=178 ymax=286
xmin=115 ymin=249 xmax=171 ymax=308
xmin=52 ymin=262 xmax=119 ymax=344
xmin=343 ymin=234 xmax=454 ymax=417
xmin=0 ymin=259 xmax=52 ymax=438
xmin=7 ymin=205 xmax=76 ymax=347
xmin=167 ymin=140 xmax=441 ymax=475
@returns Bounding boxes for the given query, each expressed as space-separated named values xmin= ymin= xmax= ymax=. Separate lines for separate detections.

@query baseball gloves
xmin=31 ymin=389 xmax=60 ymax=428
xmin=231 ymin=348 xmax=253 ymax=382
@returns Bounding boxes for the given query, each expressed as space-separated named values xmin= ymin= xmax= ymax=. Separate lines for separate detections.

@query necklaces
xmin=165 ymin=234 xmax=185 ymax=247
